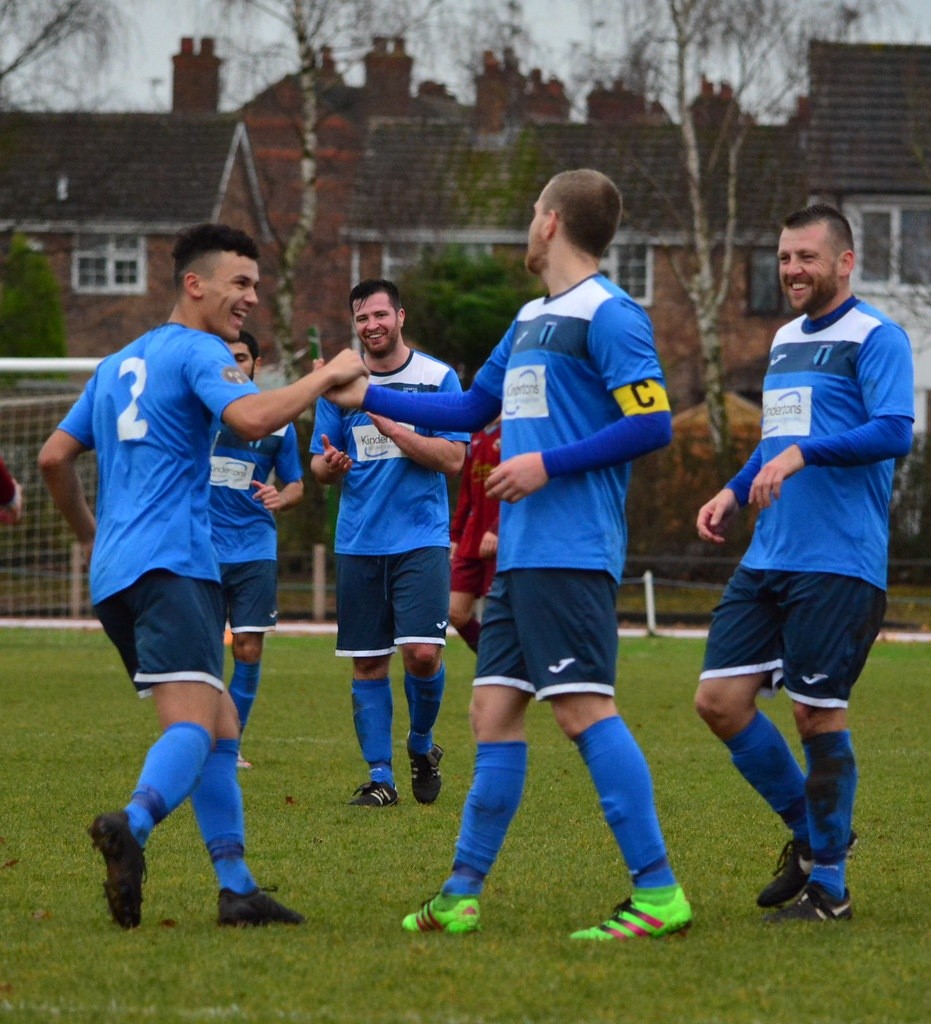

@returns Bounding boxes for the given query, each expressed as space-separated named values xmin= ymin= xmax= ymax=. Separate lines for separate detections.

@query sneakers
xmin=760 ymin=881 xmax=853 ymax=925
xmin=568 ymin=884 xmax=692 ymax=940
xmin=346 ymin=781 xmax=398 ymax=808
xmin=757 ymin=828 xmax=857 ymax=912
xmin=214 ymin=885 xmax=305 ymax=925
xmin=406 ymin=728 xmax=441 ymax=806
xmin=86 ymin=811 xmax=144 ymax=931
xmin=401 ymin=892 xmax=481 ymax=933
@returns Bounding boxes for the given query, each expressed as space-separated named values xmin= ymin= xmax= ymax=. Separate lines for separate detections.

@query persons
xmin=210 ymin=332 xmax=304 ymax=765
xmin=313 ymin=168 xmax=696 ymax=942
xmin=0 ymin=457 xmax=24 ymax=518
xmin=309 ymin=280 xmax=470 ymax=807
xmin=39 ymin=224 xmax=370 ymax=925
xmin=450 ymin=416 xmax=502 ymax=653
xmin=693 ymin=205 xmax=916 ymax=922
